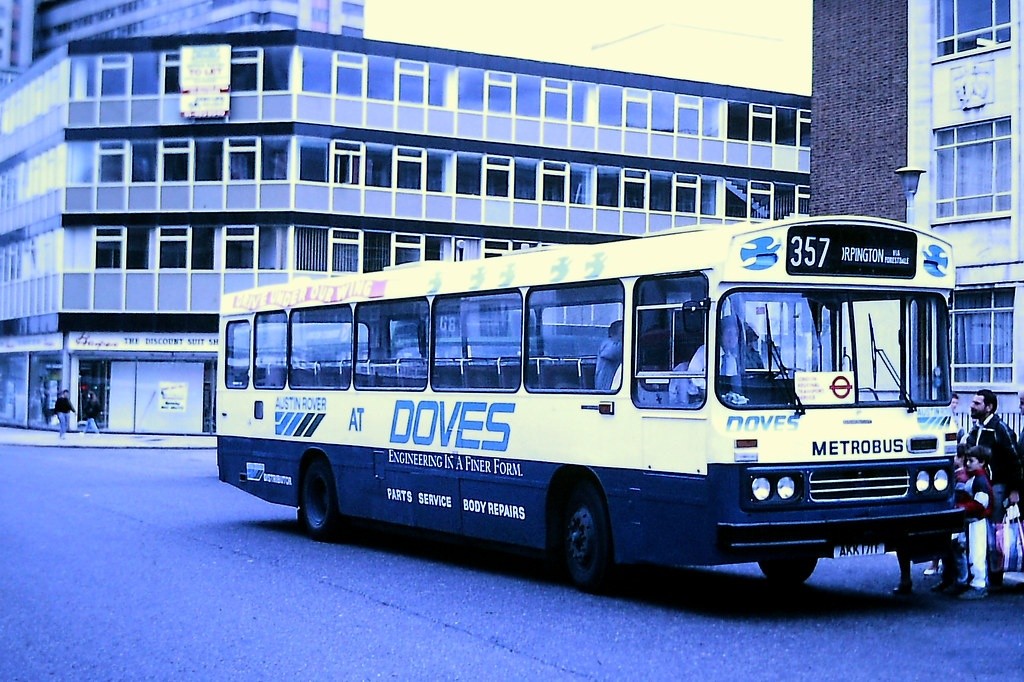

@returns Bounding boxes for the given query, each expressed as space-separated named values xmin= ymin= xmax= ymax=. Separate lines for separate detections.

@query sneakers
xmin=958 ymin=588 xmax=988 ymax=600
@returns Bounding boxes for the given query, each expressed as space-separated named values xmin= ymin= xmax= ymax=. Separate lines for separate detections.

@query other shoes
xmin=930 ymin=580 xmax=949 ymax=591
xmin=943 ymin=586 xmax=969 ymax=595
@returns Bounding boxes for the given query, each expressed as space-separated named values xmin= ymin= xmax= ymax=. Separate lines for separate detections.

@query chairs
xmin=237 ymin=356 xmax=597 ymax=388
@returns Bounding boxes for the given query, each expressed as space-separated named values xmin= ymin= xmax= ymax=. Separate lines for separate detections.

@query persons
xmin=79 ymin=391 xmax=102 ymax=436
xmin=594 ymin=320 xmax=624 ymax=391
xmin=894 ymin=388 xmax=1023 ymax=601
xmin=687 ymin=314 xmax=747 ymax=406
xmin=53 ymin=390 xmax=76 ymax=440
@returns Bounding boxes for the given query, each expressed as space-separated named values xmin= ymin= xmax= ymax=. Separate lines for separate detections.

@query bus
xmin=217 ymin=213 xmax=966 ymax=592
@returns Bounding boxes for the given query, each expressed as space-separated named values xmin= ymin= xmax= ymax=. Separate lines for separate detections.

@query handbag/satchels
xmin=986 ymin=497 xmax=1024 ymax=573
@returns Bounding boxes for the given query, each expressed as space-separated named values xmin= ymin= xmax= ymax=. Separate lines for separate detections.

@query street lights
xmin=895 ymin=166 xmax=927 ymax=224
